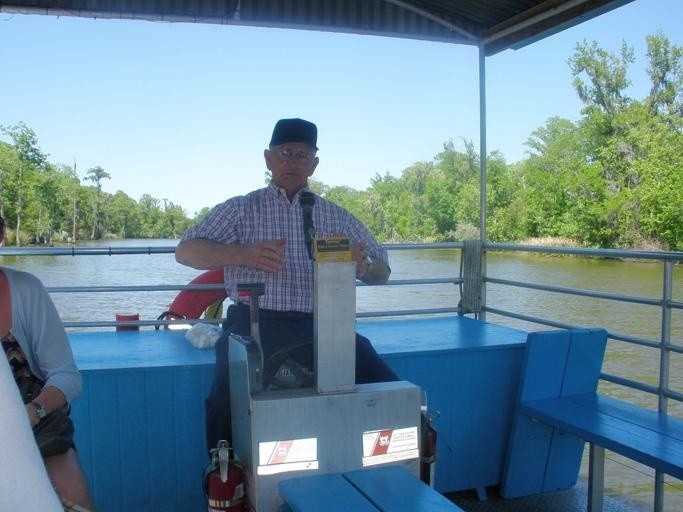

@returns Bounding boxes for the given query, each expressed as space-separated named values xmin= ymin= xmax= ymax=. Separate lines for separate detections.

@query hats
xmin=267 ymin=118 xmax=320 ymax=152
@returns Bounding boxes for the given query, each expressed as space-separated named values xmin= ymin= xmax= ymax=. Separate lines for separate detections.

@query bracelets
xmin=31 ymin=400 xmax=48 ymax=421
xmin=360 ymin=251 xmax=373 ymax=280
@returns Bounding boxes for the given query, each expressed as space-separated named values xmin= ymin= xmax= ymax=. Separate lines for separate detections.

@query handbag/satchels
xmin=32 ymin=409 xmax=76 ymax=458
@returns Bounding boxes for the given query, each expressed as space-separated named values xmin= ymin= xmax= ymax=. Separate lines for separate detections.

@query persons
xmin=0 ymin=216 xmax=94 ymax=512
xmin=173 ymin=116 xmax=438 ymax=491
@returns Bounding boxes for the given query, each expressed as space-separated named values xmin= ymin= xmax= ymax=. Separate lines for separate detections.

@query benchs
xmin=280 ymin=465 xmax=467 ymax=511
xmin=517 ymin=391 xmax=683 ymax=510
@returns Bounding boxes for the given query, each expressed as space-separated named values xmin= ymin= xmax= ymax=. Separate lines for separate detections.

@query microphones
xmin=299 ymin=191 xmax=316 ymax=259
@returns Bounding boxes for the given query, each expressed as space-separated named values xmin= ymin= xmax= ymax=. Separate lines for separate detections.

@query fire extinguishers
xmin=206 ymin=438 xmax=245 ymax=512
xmin=421 ymin=410 xmax=439 ymax=486
